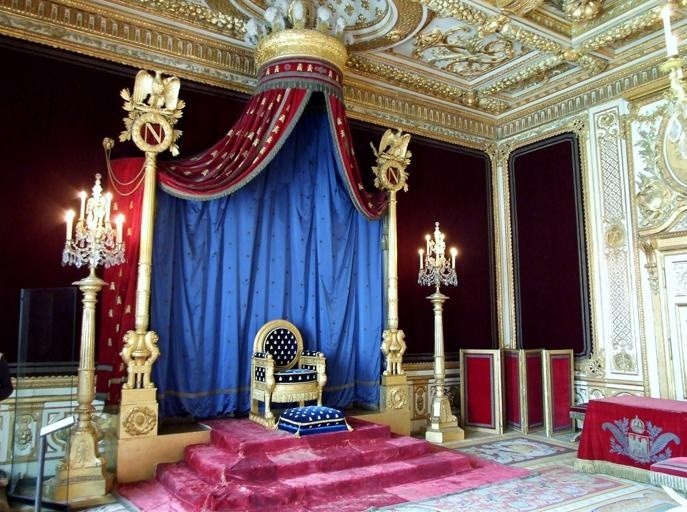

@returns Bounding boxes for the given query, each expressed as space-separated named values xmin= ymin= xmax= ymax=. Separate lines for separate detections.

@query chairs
xmin=249 ymin=319 xmax=328 ymax=419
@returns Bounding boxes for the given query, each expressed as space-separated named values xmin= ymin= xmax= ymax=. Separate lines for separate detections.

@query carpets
xmin=14 ymin=431 xmax=686 ymax=511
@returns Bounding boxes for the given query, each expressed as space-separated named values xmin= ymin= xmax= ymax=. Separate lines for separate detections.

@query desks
xmin=573 ymin=394 xmax=687 ymax=491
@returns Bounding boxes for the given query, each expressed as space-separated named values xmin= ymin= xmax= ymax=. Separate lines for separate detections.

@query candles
xmin=104 ymin=191 xmax=112 ymax=222
xmin=419 ymin=249 xmax=425 ymax=269
xmin=115 ymin=213 xmax=125 ymax=243
xmin=440 ymin=232 xmax=445 ymax=258
xmin=450 ymin=247 xmax=458 ymax=268
xmin=65 ymin=209 xmax=75 ymax=240
xmin=79 ymin=190 xmax=86 ymax=219
xmin=426 ymin=233 xmax=431 ymax=254
